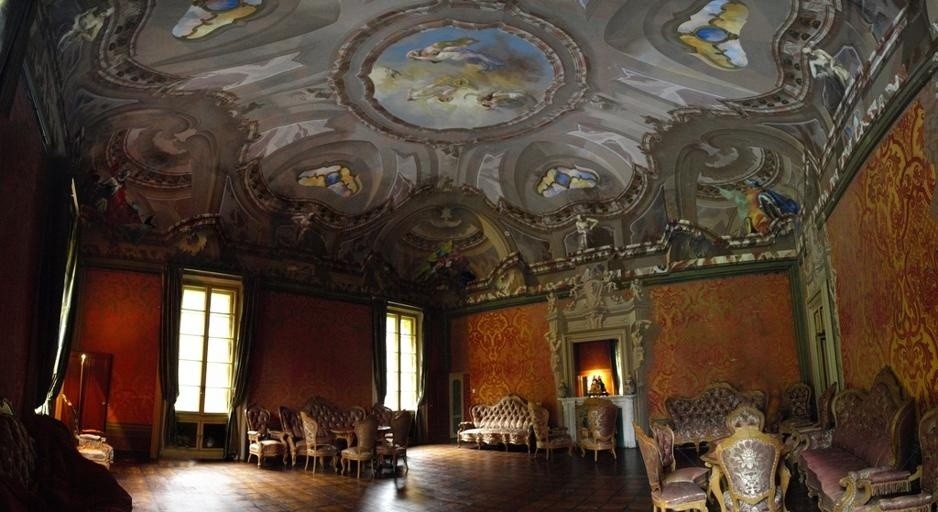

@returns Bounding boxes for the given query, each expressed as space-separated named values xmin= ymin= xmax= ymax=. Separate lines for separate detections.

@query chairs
xmin=780 ymin=380 xmax=837 ymax=484
xmin=629 ymin=417 xmax=708 ymax=512
xmin=298 ymin=404 xmax=411 ymax=476
xmin=851 ymin=409 xmax=937 ymax=512
xmin=528 ymin=401 xmax=572 ymax=460
xmin=570 ymin=398 xmax=618 ymax=459
xmin=710 ymin=407 xmax=792 ymax=512
xmin=246 ymin=406 xmax=285 ymax=468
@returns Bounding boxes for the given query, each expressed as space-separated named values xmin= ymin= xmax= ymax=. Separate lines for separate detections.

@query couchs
xmin=456 ymin=392 xmax=536 ymax=455
xmin=2 ymin=409 xmax=131 ymax=511
xmin=278 ymin=396 xmax=371 ymax=466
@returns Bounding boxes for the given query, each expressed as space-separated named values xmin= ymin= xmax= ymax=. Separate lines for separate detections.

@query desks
xmin=700 ymin=434 xmax=791 ymax=512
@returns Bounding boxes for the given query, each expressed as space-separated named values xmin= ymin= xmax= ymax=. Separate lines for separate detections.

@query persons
xmin=407 ymin=38 xmax=537 ymax=111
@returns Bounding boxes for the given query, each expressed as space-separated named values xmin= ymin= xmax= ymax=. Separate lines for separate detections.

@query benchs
xmin=797 ymin=365 xmax=914 ymax=512
xmin=663 ymin=382 xmax=768 ymax=455
